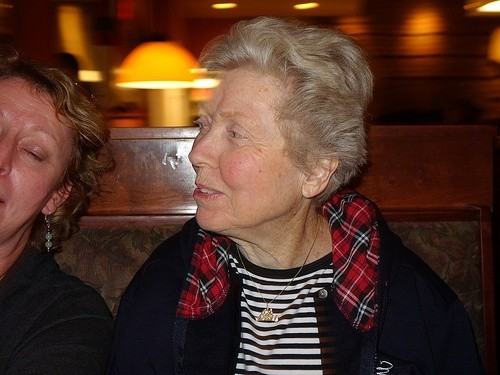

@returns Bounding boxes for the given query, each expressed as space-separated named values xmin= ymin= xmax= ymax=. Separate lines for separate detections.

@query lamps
xmin=116 ymin=33 xmax=223 ymax=91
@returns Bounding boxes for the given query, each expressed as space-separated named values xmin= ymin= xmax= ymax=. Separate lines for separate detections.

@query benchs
xmin=32 ymin=125 xmax=500 ymax=375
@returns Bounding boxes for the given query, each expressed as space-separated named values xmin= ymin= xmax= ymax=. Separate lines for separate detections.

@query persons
xmin=0 ymin=38 xmax=116 ymax=375
xmin=102 ymin=18 xmax=486 ymax=375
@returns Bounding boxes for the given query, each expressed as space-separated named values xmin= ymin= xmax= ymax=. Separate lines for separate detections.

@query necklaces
xmin=236 ymin=215 xmax=321 ymax=322
